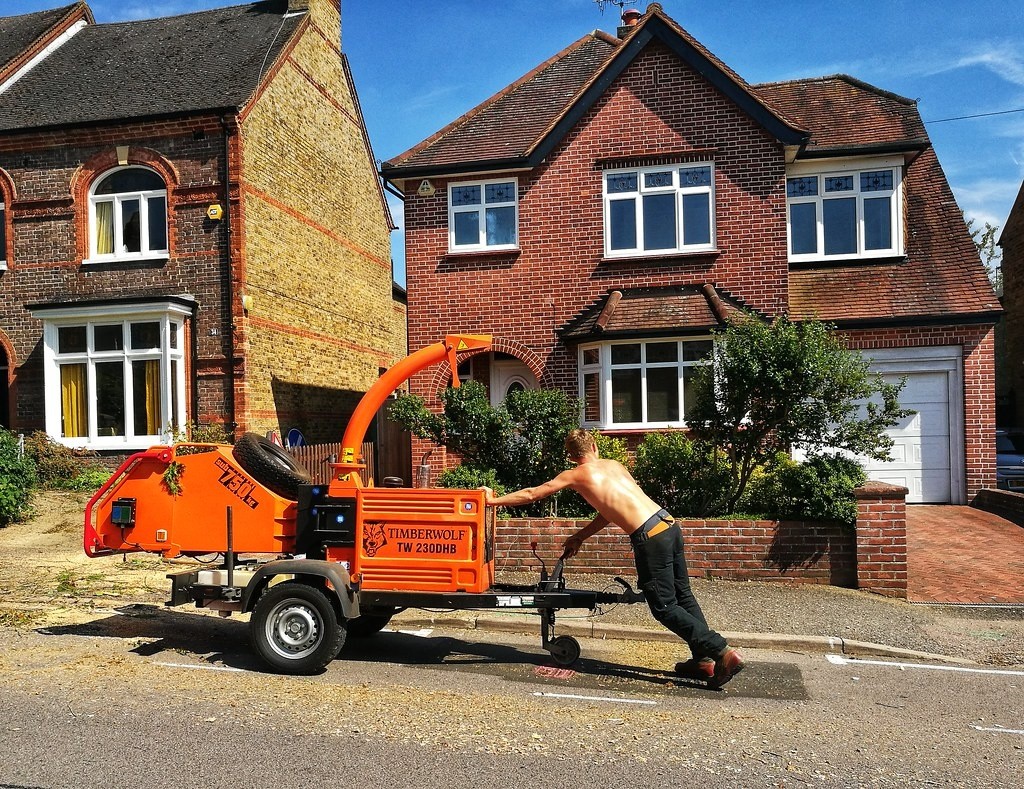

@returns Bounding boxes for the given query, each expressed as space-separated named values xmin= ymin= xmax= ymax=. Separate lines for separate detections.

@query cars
xmin=996 ymin=430 xmax=1024 ymax=494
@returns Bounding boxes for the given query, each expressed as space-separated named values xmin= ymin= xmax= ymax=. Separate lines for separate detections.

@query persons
xmin=474 ymin=428 xmax=746 ymax=690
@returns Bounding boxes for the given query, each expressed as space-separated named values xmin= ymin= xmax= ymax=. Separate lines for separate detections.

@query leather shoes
xmin=675 ymin=658 xmax=715 ymax=677
xmin=707 ymin=649 xmax=747 ymax=689
